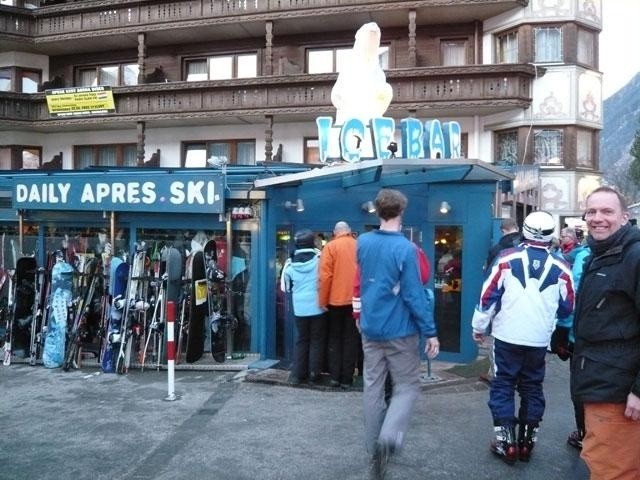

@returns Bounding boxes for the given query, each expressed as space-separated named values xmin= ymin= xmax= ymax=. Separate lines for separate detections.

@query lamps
xmin=361 ymin=200 xmax=377 ymax=213
xmin=284 ymin=198 xmax=305 ymax=212
xmin=439 ymin=201 xmax=452 ymax=215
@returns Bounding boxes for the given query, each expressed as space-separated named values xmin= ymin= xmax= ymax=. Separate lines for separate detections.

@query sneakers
xmin=288 ymin=370 xmax=354 ymax=389
xmin=569 ymin=431 xmax=584 ymax=450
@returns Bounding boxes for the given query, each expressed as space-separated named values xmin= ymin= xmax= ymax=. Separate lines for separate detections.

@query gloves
xmin=551 ymin=327 xmax=572 ymax=360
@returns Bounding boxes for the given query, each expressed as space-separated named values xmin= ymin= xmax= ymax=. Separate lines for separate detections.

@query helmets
xmin=294 ymin=230 xmax=314 ymax=248
xmin=522 ymin=212 xmax=555 ymax=242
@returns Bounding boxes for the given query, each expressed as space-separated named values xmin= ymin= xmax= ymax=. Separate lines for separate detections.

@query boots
xmin=519 ymin=420 xmax=539 ymax=461
xmin=489 ymin=418 xmax=518 ymax=465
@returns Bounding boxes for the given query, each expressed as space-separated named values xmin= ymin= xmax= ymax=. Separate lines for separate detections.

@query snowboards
xmin=11 ymin=257 xmax=38 ymax=359
xmin=101 ymin=257 xmax=129 ymax=375
xmin=42 ymin=262 xmax=74 ymax=369
xmin=162 ymin=238 xmax=238 ymax=363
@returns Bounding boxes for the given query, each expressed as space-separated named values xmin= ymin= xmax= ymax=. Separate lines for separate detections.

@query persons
xmin=479 ymin=227 xmax=591 ymax=388
xmin=356 ymin=188 xmax=441 ymax=479
xmin=471 ymin=211 xmax=575 ymax=466
xmin=573 ymin=187 xmax=640 ymax=480
xmin=279 ymin=220 xmax=462 ymax=402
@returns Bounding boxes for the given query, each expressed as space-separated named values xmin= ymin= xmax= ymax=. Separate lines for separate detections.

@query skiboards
xmin=0 ymin=233 xmax=21 ymax=365
xmin=62 ymin=253 xmax=111 ymax=372
xmin=29 ymin=250 xmax=63 ymax=366
xmin=114 ymin=242 xmax=175 ymax=375
